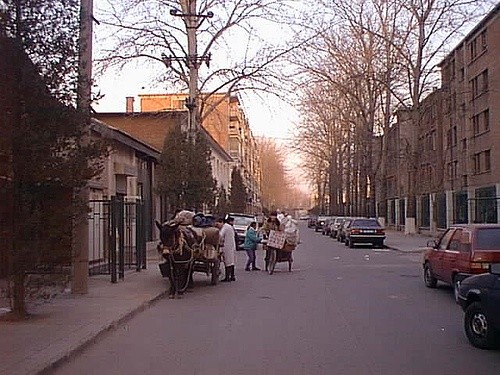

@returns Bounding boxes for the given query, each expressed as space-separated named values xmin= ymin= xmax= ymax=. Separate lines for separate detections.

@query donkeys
xmin=155 ymin=219 xmax=199 ymax=299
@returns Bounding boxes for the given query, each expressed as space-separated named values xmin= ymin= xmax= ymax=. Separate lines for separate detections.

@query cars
xmin=308 ymin=215 xmax=386 ymax=248
xmin=423 ymin=223 xmax=500 ymax=288
xmin=456 ymin=265 xmax=500 ymax=349
xmin=255 ymin=213 xmax=266 ymax=223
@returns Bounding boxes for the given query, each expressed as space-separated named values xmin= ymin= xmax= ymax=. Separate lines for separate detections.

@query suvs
xmin=225 ymin=211 xmax=259 ymax=250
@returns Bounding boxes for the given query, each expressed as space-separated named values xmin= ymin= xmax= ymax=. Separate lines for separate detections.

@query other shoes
xmin=221 ymin=279 xmax=230 ymax=282
xmin=252 ymin=267 xmax=260 ymax=270
xmin=245 ymin=268 xmax=250 ymax=271
xmin=229 ymin=277 xmax=235 ymax=281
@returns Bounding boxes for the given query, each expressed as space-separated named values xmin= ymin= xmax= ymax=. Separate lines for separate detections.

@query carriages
xmin=154 ymin=209 xmax=224 ymax=298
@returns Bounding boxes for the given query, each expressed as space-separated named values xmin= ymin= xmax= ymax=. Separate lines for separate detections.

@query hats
xmin=269 ymin=210 xmax=277 ymax=219
xmin=225 ymin=216 xmax=234 ymax=223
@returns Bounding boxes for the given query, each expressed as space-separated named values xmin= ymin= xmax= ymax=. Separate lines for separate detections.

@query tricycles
xmin=260 ymin=229 xmax=294 ymax=275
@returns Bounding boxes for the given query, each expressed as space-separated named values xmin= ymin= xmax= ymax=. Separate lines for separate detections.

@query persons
xmin=216 ymin=218 xmax=236 ymax=282
xmin=260 ymin=211 xmax=282 ymax=262
xmin=225 ymin=216 xmax=239 ymax=276
xmin=243 ymin=222 xmax=262 ymax=272
xmin=281 ymin=211 xmax=289 ymax=217
